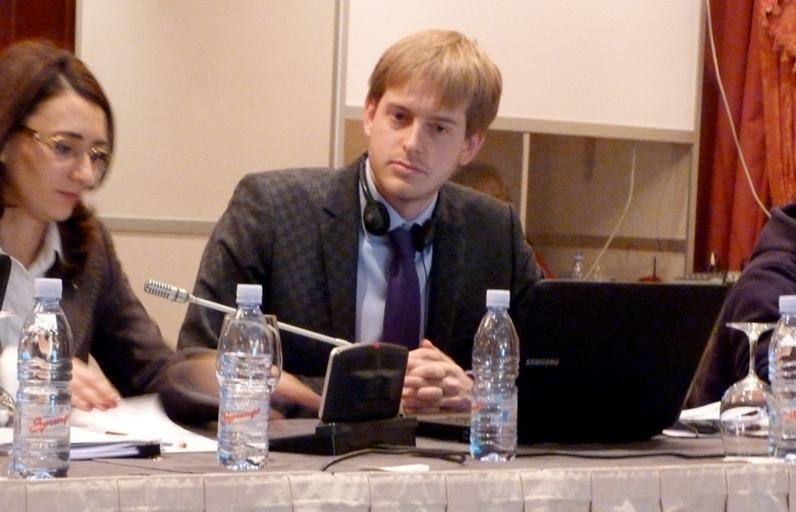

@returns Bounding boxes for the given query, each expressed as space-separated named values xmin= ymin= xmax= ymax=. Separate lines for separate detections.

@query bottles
xmin=570 ymin=251 xmax=587 ymax=279
xmin=469 ymin=287 xmax=521 ymax=466
xmin=215 ymin=285 xmax=277 ymax=473
xmin=768 ymin=294 xmax=795 ymax=459
xmin=17 ymin=278 xmax=71 ymax=480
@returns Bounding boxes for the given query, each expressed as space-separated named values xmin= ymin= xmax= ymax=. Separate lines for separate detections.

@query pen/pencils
xmin=105 ymin=431 xmax=186 ymax=448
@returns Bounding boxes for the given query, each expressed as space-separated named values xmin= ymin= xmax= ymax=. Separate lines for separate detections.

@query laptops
xmin=406 ymin=283 xmax=732 ymax=443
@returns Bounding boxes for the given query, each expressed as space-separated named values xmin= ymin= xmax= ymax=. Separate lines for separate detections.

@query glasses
xmin=20 ymin=123 xmax=110 ymax=168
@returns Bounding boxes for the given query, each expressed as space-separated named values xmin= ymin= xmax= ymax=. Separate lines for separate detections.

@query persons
xmin=686 ymin=204 xmax=795 ymax=436
xmin=0 ymin=35 xmax=322 ymax=421
xmin=455 ymin=158 xmax=559 ymax=281
xmin=173 ymin=27 xmax=545 ymax=414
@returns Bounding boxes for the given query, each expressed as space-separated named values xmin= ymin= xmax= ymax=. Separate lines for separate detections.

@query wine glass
xmin=722 ymin=321 xmax=779 ymax=458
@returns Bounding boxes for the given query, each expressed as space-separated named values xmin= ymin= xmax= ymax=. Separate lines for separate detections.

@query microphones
xmin=146 ymin=281 xmax=416 ymax=457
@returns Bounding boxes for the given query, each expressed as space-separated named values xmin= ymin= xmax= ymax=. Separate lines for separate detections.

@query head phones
xmin=353 ymin=151 xmax=446 ymax=252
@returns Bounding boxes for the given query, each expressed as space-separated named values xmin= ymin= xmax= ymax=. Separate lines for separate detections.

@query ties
xmin=383 ymin=228 xmax=421 ymax=351
